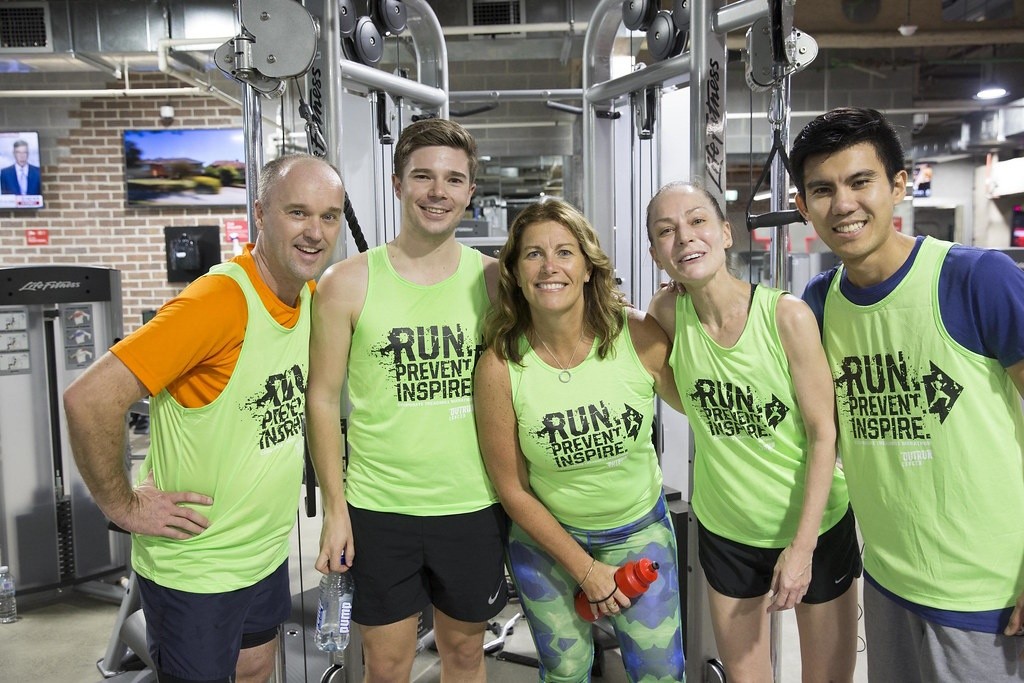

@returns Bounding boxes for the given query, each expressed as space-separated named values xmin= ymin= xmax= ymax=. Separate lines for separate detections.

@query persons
xmin=473 ymin=197 xmax=686 ymax=683
xmin=646 ymin=181 xmax=863 ymax=683
xmin=62 ymin=154 xmax=344 ymax=683
xmin=0 ymin=140 xmax=40 ymax=196
xmin=659 ymin=108 xmax=1023 ymax=683
xmin=306 ymin=120 xmax=500 ymax=683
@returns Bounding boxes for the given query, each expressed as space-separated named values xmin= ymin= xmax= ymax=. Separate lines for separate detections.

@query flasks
xmin=576 ymin=558 xmax=659 ymax=621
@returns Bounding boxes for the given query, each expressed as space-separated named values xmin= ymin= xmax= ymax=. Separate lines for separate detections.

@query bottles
xmin=313 ymin=556 xmax=355 ymax=653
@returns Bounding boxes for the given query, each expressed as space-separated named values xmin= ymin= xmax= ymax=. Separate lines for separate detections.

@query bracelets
xmin=579 ymin=559 xmax=596 ymax=587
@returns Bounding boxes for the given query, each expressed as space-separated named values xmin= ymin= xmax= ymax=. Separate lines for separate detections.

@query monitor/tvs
xmin=0 ymin=129 xmax=45 ymax=211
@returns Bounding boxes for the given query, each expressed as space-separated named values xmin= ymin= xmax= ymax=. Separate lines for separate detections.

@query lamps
xmin=976 ymin=44 xmax=1006 ymax=100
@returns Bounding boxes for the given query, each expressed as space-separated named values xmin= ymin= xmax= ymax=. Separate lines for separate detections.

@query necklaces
xmin=533 ymin=324 xmax=587 ymax=382
